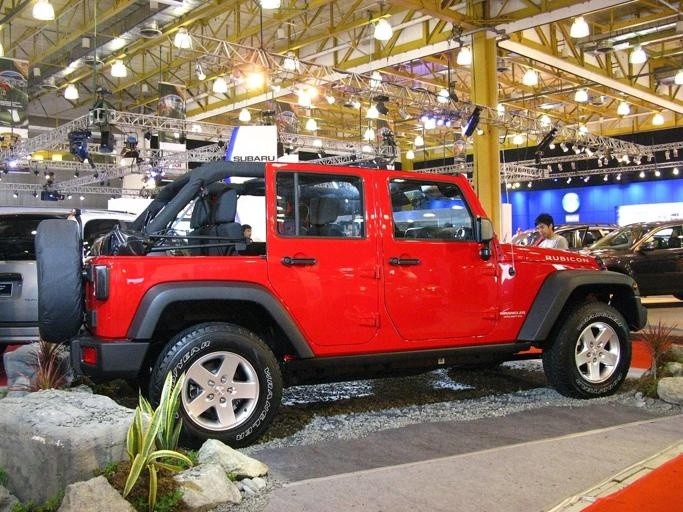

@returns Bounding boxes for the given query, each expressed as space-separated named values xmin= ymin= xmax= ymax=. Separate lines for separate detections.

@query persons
xmin=534 ymin=212 xmax=569 ymax=250
xmin=513 ymin=227 xmax=528 ymax=246
xmin=241 ymin=224 xmax=252 ymax=239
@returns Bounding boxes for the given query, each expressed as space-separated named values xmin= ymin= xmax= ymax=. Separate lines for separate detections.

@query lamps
xmin=31 ymin=1 xmax=683 ymax=184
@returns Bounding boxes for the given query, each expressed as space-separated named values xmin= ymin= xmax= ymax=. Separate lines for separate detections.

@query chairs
xmin=189 ymin=189 xmax=252 ymax=257
xmin=281 ymin=188 xmax=343 ymax=234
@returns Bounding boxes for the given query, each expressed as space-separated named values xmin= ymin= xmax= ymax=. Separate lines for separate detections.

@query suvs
xmin=32 ymin=158 xmax=650 ymax=451
xmin=0 ymin=208 xmax=189 ymax=349
xmin=332 ymin=263 xmax=450 ymax=328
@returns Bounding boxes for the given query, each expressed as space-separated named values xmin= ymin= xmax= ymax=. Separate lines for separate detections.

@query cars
xmin=508 ymin=218 xmax=682 ymax=308
